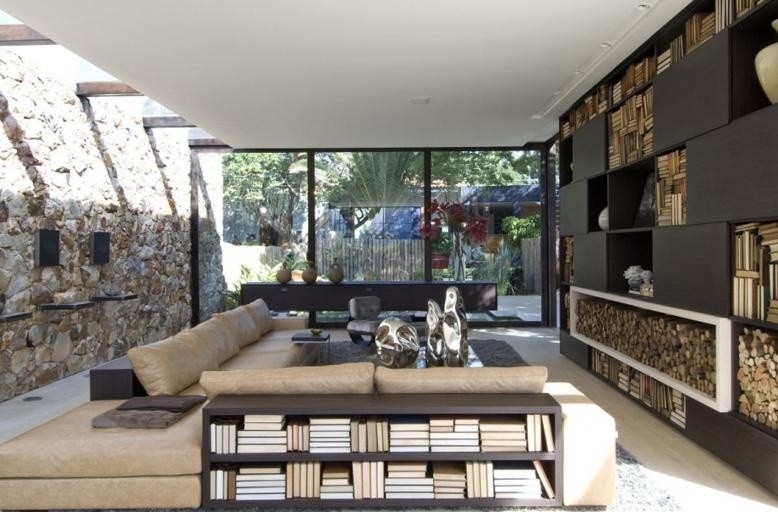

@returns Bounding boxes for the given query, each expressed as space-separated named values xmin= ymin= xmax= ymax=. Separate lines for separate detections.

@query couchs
xmin=0 ymin=399 xmax=202 ymax=509
xmin=90 ymin=317 xmax=309 ymax=402
xmin=544 ymin=381 xmax=616 ymax=507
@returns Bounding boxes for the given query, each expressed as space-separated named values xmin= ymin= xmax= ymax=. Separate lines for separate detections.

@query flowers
xmin=420 ymin=199 xmax=489 ymax=243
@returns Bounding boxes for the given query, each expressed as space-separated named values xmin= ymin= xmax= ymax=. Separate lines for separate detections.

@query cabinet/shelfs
xmin=200 ymin=394 xmax=565 ymax=509
xmin=240 ymin=281 xmax=497 ymax=329
xmin=559 ymin=0 xmax=778 ymax=496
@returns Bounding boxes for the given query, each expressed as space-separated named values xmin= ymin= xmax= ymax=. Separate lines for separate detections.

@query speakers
xmin=90 ymin=231 xmax=110 ymax=265
xmin=34 ymin=229 xmax=59 ymax=267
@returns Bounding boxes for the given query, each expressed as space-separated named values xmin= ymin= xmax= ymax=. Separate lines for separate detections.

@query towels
xmin=115 ymin=395 xmax=205 ymax=413
xmin=91 ymin=409 xmax=183 ymax=429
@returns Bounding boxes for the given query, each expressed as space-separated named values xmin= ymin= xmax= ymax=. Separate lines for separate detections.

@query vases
xmin=754 ymin=19 xmax=778 ymax=104
xmin=453 ymin=232 xmax=467 ymax=282
xmin=276 ymin=257 xmax=344 ymax=283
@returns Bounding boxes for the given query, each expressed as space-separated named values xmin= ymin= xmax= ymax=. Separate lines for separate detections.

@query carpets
xmin=192 ymin=339 xmax=679 ymax=512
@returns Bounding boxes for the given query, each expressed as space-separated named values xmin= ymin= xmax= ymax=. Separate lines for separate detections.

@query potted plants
xmin=431 ymin=232 xmax=450 ymax=268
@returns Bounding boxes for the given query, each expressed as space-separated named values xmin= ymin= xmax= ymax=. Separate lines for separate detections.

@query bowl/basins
xmin=312 ymin=329 xmax=321 ymax=335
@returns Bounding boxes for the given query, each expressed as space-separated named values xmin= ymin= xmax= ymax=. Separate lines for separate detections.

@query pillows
xmin=374 ymin=365 xmax=548 ymax=394
xmin=198 ymin=360 xmax=374 ymax=396
xmin=126 ymin=298 xmax=274 ymax=397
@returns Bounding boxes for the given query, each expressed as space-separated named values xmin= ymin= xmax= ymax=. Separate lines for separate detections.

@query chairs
xmin=345 ymin=296 xmax=383 ymax=347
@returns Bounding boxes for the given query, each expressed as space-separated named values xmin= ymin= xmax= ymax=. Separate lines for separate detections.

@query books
xmin=210 ymin=414 xmax=555 ymax=454
xmin=729 ymin=221 xmax=778 ymax=323
xmin=553 ymin=1 xmax=773 ymax=169
xmin=590 ymin=354 xmax=688 ymax=431
xmin=210 ymin=461 xmax=555 ymax=501
xmin=655 ymin=149 xmax=687 ymax=227
